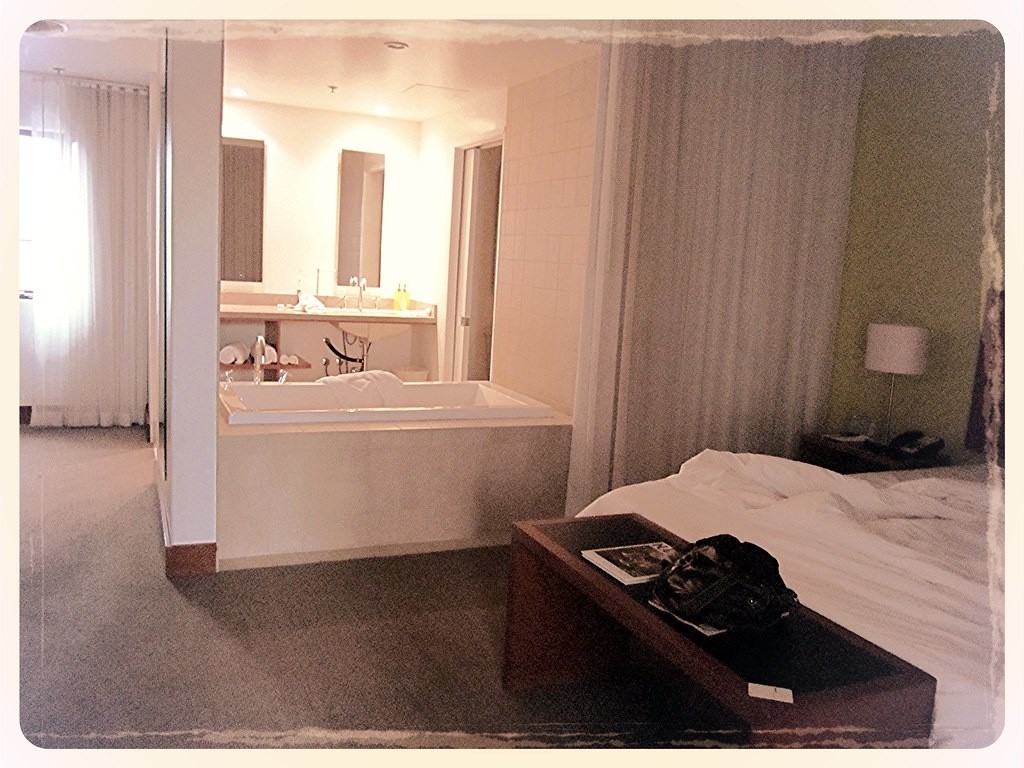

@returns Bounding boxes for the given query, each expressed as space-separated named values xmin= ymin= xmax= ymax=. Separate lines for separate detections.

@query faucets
xmin=349 ymin=276 xmax=362 ymax=290
xmin=251 ymin=335 xmax=269 ymax=386
xmin=358 ymin=277 xmax=370 ymax=312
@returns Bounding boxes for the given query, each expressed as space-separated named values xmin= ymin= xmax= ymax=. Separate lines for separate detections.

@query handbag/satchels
xmin=651 ymin=533 xmax=801 ymax=633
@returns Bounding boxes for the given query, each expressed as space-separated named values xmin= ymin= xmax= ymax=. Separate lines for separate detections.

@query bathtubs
xmin=211 ymin=370 xmax=579 ymax=574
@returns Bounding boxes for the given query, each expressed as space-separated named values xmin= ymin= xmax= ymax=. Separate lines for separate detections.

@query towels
xmin=315 ymin=371 xmax=410 ymax=411
xmin=249 ymin=344 xmax=279 ymax=367
xmin=278 ymin=351 xmax=289 ymax=365
xmin=292 ymin=293 xmax=328 ymax=317
xmin=219 ymin=340 xmax=251 ymax=367
xmin=288 ymin=353 xmax=301 ymax=366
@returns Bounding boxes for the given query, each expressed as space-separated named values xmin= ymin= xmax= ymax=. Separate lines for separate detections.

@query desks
xmin=501 ymin=510 xmax=937 ymax=748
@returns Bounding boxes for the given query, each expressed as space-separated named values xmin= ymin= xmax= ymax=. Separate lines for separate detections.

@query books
xmin=648 ymin=598 xmax=791 ymax=638
xmin=580 ymin=541 xmax=685 ymax=585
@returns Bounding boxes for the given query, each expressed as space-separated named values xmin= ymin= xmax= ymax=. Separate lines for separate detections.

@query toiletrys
xmin=399 ymin=281 xmax=411 ymax=312
xmin=391 ymin=282 xmax=404 ymax=312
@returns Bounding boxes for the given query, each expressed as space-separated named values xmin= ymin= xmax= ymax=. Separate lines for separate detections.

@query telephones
xmin=888 ymin=430 xmax=946 ymax=458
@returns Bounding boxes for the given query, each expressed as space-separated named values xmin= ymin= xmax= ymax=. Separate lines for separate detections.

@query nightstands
xmin=809 ymin=432 xmax=939 ymax=474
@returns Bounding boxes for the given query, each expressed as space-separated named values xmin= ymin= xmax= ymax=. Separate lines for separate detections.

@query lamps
xmin=864 ymin=320 xmax=931 ymax=455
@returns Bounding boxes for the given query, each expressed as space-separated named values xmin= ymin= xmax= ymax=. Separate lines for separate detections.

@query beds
xmin=573 ymin=448 xmax=1004 ymax=748
